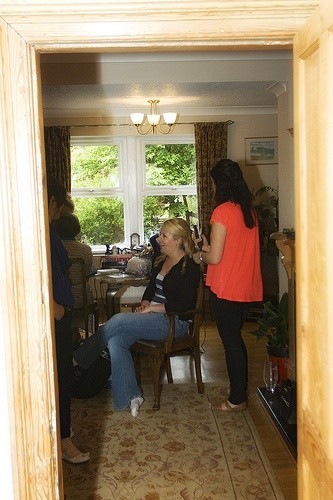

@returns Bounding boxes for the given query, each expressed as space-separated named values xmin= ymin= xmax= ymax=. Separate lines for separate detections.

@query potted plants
xmin=246 ymin=293 xmax=290 ymax=388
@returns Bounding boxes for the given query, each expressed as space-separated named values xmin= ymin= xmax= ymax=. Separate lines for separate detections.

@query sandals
xmin=210 ymin=401 xmax=247 ymax=412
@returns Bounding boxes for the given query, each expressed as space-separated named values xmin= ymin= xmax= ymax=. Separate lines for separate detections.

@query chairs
xmin=131 ymin=270 xmax=205 ymax=411
xmin=68 ymin=258 xmax=100 ymax=338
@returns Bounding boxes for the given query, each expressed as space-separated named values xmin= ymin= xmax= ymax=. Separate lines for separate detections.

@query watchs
xmin=198 ymin=252 xmax=203 ymax=261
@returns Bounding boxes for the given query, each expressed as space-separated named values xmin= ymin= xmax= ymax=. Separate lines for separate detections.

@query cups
xmin=263 ymin=359 xmax=280 ymax=394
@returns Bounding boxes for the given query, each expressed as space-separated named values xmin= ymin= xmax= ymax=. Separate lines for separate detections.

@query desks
xmin=88 ymin=253 xmax=147 ymax=321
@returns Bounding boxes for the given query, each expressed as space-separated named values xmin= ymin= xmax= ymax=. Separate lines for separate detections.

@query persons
xmin=52 ymin=187 xmax=79 ymax=239
xmin=72 ymin=219 xmax=203 ymax=418
xmin=194 ymin=158 xmax=263 ymax=413
xmin=46 ymin=175 xmax=92 ymax=464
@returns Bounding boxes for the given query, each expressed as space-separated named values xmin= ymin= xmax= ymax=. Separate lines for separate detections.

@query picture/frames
xmin=245 ymin=136 xmax=278 ymax=165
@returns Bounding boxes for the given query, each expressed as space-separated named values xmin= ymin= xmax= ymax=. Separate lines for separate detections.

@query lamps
xmin=130 ymin=101 xmax=179 ymax=135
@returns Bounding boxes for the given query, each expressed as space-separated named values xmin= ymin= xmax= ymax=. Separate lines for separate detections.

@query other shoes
xmin=62 ymin=452 xmax=90 ymax=464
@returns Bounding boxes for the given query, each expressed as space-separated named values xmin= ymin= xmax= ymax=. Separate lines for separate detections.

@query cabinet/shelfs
xmin=256 ymin=226 xmax=296 ymax=463
